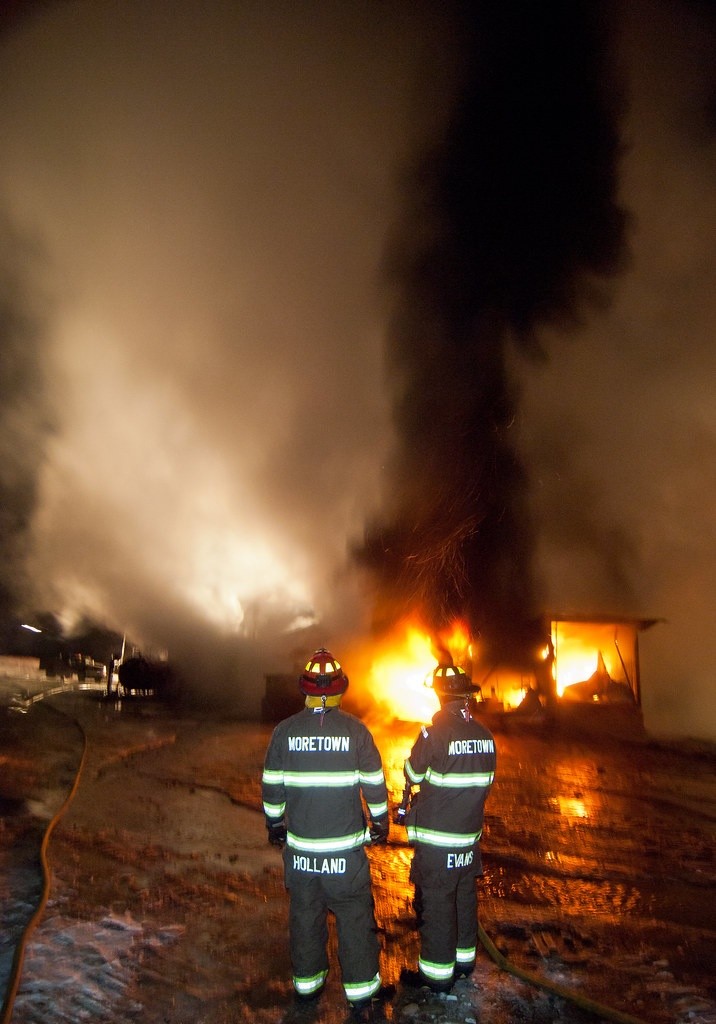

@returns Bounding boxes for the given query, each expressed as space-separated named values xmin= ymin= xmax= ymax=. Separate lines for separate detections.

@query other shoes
xmin=400 ymin=956 xmax=477 ymax=990
xmin=296 ymin=979 xmax=396 ymax=1009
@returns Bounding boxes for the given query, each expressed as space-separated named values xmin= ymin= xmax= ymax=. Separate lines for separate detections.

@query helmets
xmin=425 ymin=664 xmax=480 ymax=694
xmin=299 ymin=648 xmax=348 ymax=697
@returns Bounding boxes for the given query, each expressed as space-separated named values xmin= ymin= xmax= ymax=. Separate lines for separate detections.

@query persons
xmin=261 ymin=648 xmax=396 ymax=1009
xmin=400 ymin=664 xmax=497 ymax=987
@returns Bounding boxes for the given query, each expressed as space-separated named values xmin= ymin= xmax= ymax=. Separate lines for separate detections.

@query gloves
xmin=369 ymin=819 xmax=389 ymax=845
xmin=268 ymin=824 xmax=287 ymax=848
